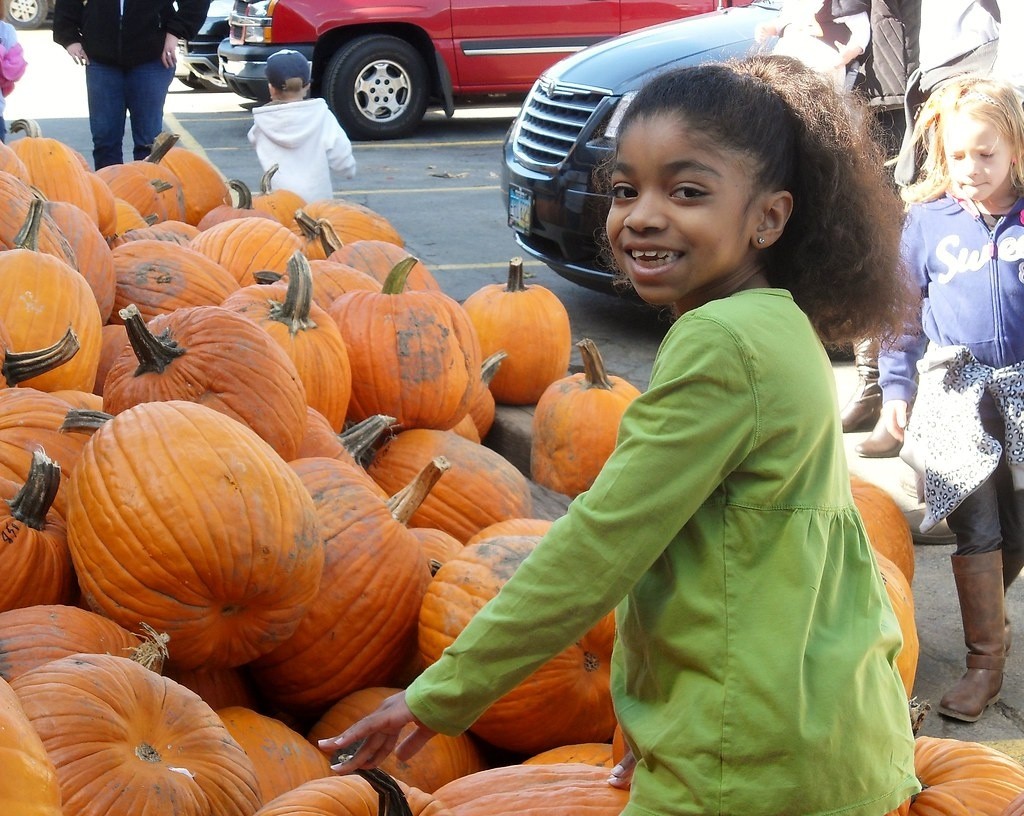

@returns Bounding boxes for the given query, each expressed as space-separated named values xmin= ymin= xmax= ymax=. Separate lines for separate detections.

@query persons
xmin=318 ymin=55 xmax=922 ymax=816
xmin=0 ymin=0 xmax=28 ymax=144
xmin=53 ymin=0 xmax=211 ymax=171
xmin=754 ymin=0 xmax=1003 ymax=457
xmin=248 ymin=50 xmax=357 ymax=203
xmin=877 ymin=78 xmax=1024 ymax=722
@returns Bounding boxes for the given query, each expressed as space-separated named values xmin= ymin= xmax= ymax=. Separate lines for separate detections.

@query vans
xmin=217 ymin=0 xmax=756 ymax=141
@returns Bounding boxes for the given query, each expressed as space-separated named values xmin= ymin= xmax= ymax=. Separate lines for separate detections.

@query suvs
xmin=174 ymin=0 xmax=236 ymax=93
xmin=502 ymin=0 xmax=794 ymax=301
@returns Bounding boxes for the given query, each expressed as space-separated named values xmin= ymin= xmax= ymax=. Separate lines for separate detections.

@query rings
xmin=167 ymin=51 xmax=171 ymax=56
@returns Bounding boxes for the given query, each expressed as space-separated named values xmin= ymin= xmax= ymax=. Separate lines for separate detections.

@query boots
xmin=937 ymin=549 xmax=1023 ymax=722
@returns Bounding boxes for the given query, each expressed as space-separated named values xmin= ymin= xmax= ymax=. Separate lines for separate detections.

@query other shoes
xmin=856 ymin=403 xmax=910 ymax=457
xmin=905 ymin=516 xmax=959 ymax=545
xmin=838 ymin=379 xmax=883 ymax=430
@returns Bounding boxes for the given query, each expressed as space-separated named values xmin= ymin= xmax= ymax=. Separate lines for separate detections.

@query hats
xmin=265 ymin=49 xmax=310 ymax=90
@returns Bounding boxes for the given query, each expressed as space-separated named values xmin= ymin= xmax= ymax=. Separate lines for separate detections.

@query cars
xmin=0 ymin=0 xmax=57 ymax=31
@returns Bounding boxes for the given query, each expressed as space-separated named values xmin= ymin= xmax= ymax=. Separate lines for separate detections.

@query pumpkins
xmin=0 ymin=119 xmax=1024 ymax=816
